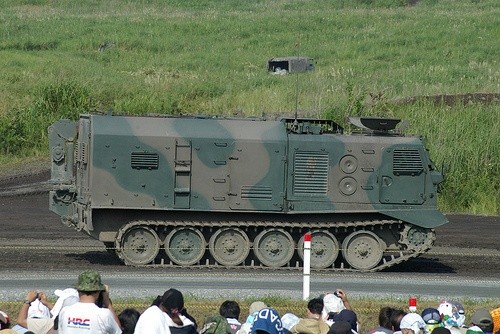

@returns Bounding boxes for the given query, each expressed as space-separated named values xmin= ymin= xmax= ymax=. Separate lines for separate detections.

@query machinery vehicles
xmin=47 ymin=113 xmax=449 ymax=274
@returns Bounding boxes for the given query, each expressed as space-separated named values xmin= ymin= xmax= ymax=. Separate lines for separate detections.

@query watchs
xmin=23 ymin=300 xmax=31 ymax=306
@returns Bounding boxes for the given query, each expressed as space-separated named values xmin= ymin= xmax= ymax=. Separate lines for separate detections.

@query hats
xmin=400 ymin=313 xmax=429 ymax=334
xmin=73 ymin=269 xmax=106 ymax=291
xmin=249 ymin=301 xmax=267 ymax=314
xmin=472 ymin=309 xmax=493 ymax=327
xmin=55 ymin=288 xmax=79 ymax=306
xmin=332 ymin=309 xmax=357 ymax=328
xmin=323 ymin=294 xmax=346 ymax=315
xmin=289 ymin=319 xmax=330 ymax=334
xmin=422 ymin=308 xmax=441 ymax=324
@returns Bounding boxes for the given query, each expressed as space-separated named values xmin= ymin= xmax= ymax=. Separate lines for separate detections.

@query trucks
xmin=267 ymin=56 xmax=318 ymax=75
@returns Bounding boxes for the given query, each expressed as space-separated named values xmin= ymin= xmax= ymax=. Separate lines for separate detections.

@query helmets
xmin=437 ymin=301 xmax=467 ymax=328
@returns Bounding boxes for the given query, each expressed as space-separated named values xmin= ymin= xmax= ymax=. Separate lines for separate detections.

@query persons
xmin=58 ymin=271 xmax=124 ymax=334
xmin=0 ymin=270 xmax=500 ymax=334
xmin=199 ymin=300 xmax=243 ymax=334
